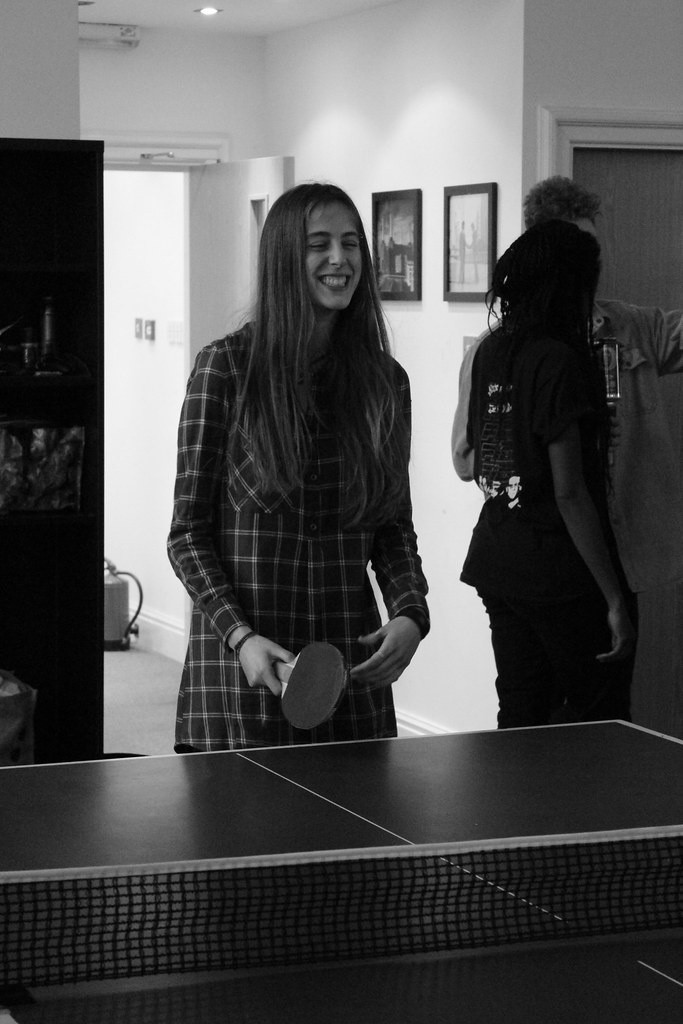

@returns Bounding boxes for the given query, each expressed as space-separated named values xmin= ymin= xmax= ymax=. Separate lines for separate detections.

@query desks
xmin=0 ymin=721 xmax=683 ymax=1024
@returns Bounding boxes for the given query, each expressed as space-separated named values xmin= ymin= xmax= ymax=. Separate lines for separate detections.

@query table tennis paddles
xmin=272 ymin=640 xmax=350 ymax=731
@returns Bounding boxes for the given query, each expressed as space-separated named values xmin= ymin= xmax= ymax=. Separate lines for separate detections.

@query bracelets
xmin=234 ymin=631 xmax=254 ymax=655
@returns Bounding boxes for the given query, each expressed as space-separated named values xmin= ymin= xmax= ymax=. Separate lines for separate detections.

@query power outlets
xmin=134 ymin=320 xmax=142 ymax=337
xmin=145 ymin=321 xmax=155 ymax=338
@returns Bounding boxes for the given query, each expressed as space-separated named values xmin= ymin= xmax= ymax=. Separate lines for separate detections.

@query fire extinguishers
xmin=104 ymin=556 xmax=144 ymax=652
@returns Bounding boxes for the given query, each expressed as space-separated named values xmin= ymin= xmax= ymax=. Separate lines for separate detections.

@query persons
xmin=451 ymin=177 xmax=683 ymax=730
xmin=459 ymin=219 xmax=642 ymax=731
xmin=167 ymin=184 xmax=431 ymax=751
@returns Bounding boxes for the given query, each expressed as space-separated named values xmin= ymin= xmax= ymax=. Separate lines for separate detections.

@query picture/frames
xmin=441 ymin=181 xmax=496 ymax=304
xmin=371 ymin=187 xmax=421 ymax=303
